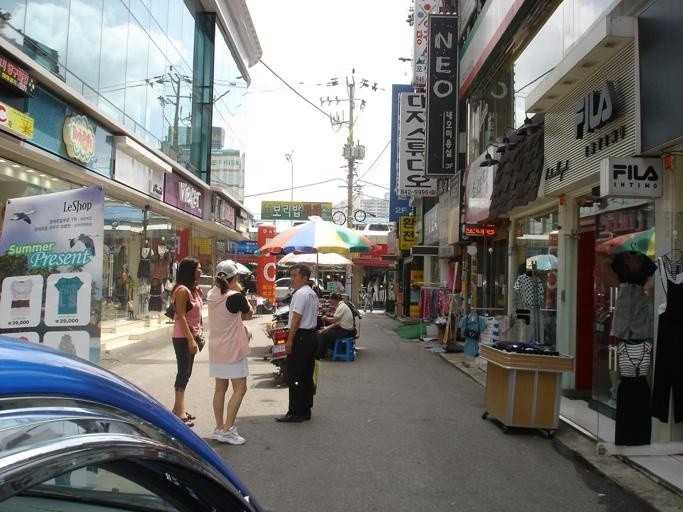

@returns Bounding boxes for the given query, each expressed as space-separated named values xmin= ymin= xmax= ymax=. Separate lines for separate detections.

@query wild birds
xmin=10 ymin=210 xmax=37 ymax=224
xmin=69 ymin=233 xmax=99 ymax=257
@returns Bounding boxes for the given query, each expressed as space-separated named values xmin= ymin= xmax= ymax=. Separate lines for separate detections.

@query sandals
xmin=171 ymin=410 xmax=195 ymax=427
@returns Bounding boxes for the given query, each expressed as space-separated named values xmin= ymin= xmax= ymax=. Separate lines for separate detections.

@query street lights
xmin=285 ymin=149 xmax=294 ymax=202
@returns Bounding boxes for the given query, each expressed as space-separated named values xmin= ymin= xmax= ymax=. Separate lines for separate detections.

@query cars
xmin=252 ymin=222 xmax=274 ymax=232
xmin=274 ymin=277 xmax=324 ymax=300
xmin=0 ymin=334 xmax=265 ymax=512
xmin=198 ymin=275 xmax=217 ymax=301
xmin=363 ymin=223 xmax=390 ymax=234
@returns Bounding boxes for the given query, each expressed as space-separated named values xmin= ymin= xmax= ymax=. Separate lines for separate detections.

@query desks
xmin=480 ymin=342 xmax=574 ymax=438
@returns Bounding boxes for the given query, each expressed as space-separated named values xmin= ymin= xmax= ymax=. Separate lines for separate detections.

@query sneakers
xmin=212 ymin=426 xmax=246 ymax=444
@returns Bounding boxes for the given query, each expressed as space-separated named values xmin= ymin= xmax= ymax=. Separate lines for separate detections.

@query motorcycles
xmin=268 ymin=304 xmax=292 ymax=384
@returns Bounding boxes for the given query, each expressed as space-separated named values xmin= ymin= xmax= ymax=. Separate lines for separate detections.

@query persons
xmin=112 ymin=264 xmax=176 ymax=324
xmin=315 ymin=292 xmax=355 ymax=359
xmin=363 ymin=280 xmax=375 ymax=312
xmin=171 ymin=256 xmax=253 ymax=445
xmin=275 ymin=264 xmax=319 ymax=422
xmin=137 ymin=236 xmax=172 ymax=283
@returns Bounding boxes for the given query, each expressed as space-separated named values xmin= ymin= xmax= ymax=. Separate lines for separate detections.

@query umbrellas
xmin=254 ymin=221 xmax=382 ymax=295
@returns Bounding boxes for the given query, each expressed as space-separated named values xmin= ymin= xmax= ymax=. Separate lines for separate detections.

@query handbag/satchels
xmin=194 ymin=335 xmax=205 ymax=352
xmin=164 ymin=302 xmax=193 ymax=320
xmin=312 ymin=360 xmax=320 ymax=395
xmin=301 ymin=340 xmax=325 ymax=359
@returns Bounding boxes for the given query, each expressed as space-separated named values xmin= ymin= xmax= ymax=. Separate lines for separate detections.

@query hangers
xmin=653 ymin=237 xmax=683 ymax=264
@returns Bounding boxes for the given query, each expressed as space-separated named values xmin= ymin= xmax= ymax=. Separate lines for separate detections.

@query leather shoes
xmin=274 ymin=415 xmax=311 ymax=422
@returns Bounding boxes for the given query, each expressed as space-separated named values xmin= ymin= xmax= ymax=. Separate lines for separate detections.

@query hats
xmin=215 ymin=260 xmax=239 ymax=279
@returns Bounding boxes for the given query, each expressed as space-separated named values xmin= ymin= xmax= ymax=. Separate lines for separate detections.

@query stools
xmin=332 ymin=337 xmax=354 ymax=362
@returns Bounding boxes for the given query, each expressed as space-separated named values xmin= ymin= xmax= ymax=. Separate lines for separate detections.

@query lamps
xmin=480 ymin=113 xmax=540 ymax=168
